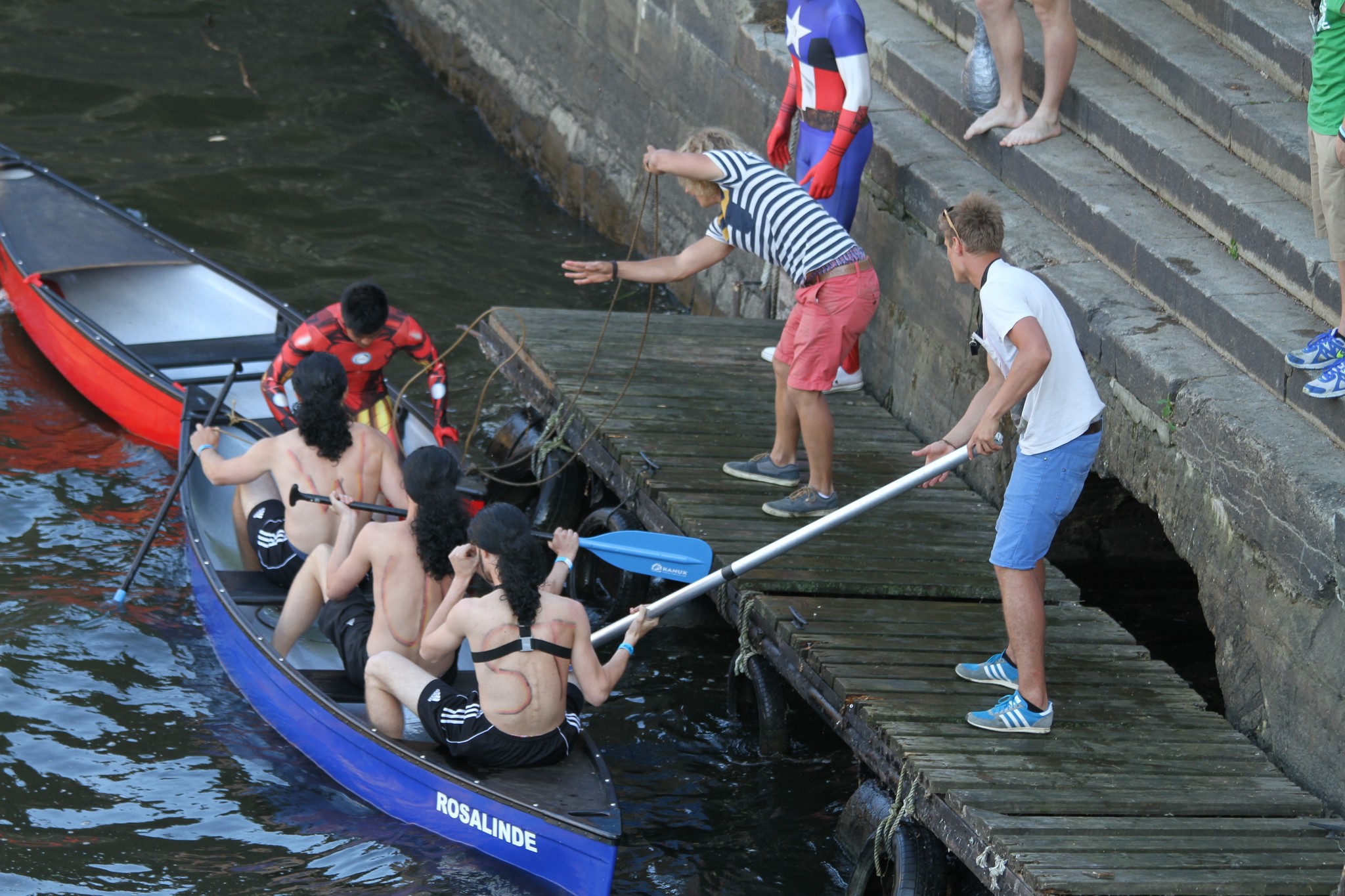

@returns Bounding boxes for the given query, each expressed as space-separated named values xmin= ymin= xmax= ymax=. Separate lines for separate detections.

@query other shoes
xmin=760 ymin=346 xmax=777 ymax=363
xmin=821 ymin=366 xmax=864 ymax=395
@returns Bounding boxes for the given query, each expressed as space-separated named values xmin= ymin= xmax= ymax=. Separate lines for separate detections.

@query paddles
xmin=288 ymin=482 xmax=713 ymax=584
xmin=102 ymin=357 xmax=243 ymax=606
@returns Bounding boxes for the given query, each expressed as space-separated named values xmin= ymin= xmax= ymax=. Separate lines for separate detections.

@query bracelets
xmin=941 ymin=439 xmax=958 ymax=451
xmin=197 ymin=445 xmax=216 ymax=455
xmin=616 ymin=642 xmax=634 ymax=656
xmin=1338 ymin=125 xmax=1345 ymax=143
xmin=555 ymin=556 xmax=574 ymax=574
xmin=609 ymin=260 xmax=617 ymax=282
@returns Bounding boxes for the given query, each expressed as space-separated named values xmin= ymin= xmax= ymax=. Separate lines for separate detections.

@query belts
xmin=802 ymin=259 xmax=872 ymax=288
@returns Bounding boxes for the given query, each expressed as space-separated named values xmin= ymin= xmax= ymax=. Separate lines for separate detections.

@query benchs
xmin=104 ymin=331 xmax=408 ymax=448
xmin=215 ymin=568 xmax=479 ymax=702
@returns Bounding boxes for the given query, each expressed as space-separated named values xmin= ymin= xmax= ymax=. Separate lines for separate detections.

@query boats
xmin=179 ymin=382 xmax=622 ymax=896
xmin=0 ymin=145 xmax=487 ymax=523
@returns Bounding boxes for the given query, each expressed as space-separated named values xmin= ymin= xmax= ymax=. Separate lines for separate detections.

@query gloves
xmin=799 ymin=150 xmax=841 ymax=201
xmin=433 ymin=417 xmax=459 ymax=449
xmin=767 ymin=109 xmax=794 ymax=169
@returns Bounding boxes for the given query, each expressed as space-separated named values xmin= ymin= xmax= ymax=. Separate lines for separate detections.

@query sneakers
xmin=965 ymin=689 xmax=1054 ymax=734
xmin=955 ymin=649 xmax=1019 ymax=690
xmin=1302 ymin=356 xmax=1345 ymax=399
xmin=1284 ymin=327 xmax=1345 ymax=371
xmin=762 ymin=485 xmax=839 ymax=518
xmin=723 ymin=450 xmax=800 ymax=487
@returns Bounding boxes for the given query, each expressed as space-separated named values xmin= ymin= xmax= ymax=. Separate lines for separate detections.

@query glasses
xmin=942 ymin=205 xmax=963 ymax=245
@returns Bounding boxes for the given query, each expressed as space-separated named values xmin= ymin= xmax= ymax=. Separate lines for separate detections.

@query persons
xmin=963 ymin=0 xmax=1075 ymax=148
xmin=1284 ymin=0 xmax=1345 ymax=403
xmin=188 ymin=282 xmax=660 ymax=771
xmin=912 ymin=192 xmax=1105 ymax=732
xmin=560 ymin=125 xmax=880 ymax=520
xmin=762 ymin=0 xmax=875 ymax=391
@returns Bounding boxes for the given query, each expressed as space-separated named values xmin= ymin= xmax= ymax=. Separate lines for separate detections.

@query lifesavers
xmin=488 ymin=445 xmax=590 ymax=546
xmin=568 ymin=509 xmax=644 ymax=631
xmin=729 ymin=656 xmax=795 ymax=762
xmin=847 ymin=817 xmax=948 ymax=896
